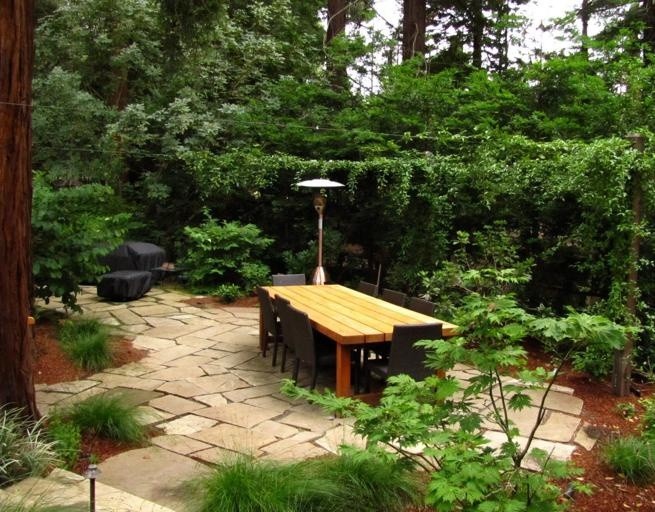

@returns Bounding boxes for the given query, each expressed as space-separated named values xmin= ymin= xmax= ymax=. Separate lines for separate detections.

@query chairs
xmin=256 ymin=273 xmax=442 ymax=404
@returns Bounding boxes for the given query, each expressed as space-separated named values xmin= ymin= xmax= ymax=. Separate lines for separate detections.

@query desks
xmin=262 ymin=284 xmax=460 ymax=419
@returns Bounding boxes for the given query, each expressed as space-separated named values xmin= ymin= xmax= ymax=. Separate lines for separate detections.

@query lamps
xmin=296 ymin=179 xmax=346 ymax=285
